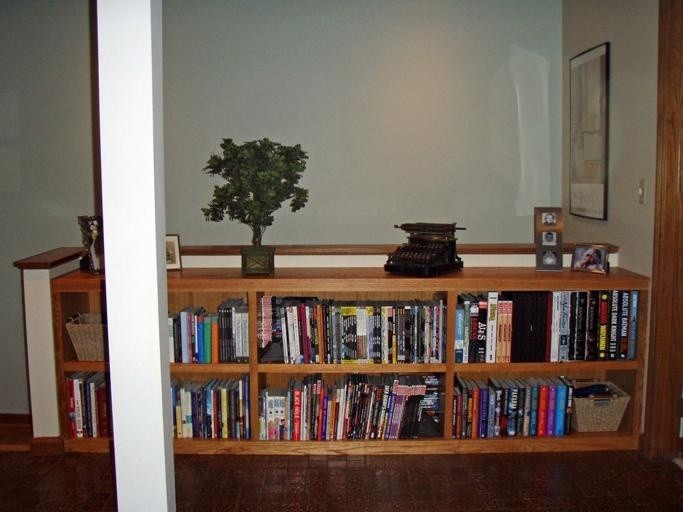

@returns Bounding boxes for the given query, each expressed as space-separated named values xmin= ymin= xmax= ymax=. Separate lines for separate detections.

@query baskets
xmin=62 ymin=310 xmax=106 ymax=364
xmin=567 ymin=379 xmax=632 ymax=433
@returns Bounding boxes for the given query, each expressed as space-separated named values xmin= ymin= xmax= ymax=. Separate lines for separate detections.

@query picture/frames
xmin=166 ymin=234 xmax=183 ymax=271
xmin=571 ymin=244 xmax=610 ymax=275
xmin=569 ymin=41 xmax=610 ymax=221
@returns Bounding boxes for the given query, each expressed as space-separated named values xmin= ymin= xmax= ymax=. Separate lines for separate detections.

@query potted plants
xmin=201 ymin=137 xmax=309 ymax=274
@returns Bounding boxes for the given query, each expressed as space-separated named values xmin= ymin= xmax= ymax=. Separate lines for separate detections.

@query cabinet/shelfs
xmin=51 ymin=268 xmax=651 ymax=456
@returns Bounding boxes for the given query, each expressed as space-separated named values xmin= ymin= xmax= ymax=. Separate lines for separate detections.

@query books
xmin=453 ymin=372 xmax=573 ymax=441
xmin=257 ymin=372 xmax=446 ymax=442
xmin=172 ymin=371 xmax=251 ymax=441
xmin=455 ymin=288 xmax=641 ymax=364
xmin=259 ymin=294 xmax=447 ymax=363
xmin=67 ymin=372 xmax=109 ymax=439
xmin=167 ymin=299 xmax=249 ymax=364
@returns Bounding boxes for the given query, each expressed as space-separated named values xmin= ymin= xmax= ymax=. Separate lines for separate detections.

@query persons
xmin=581 ymin=252 xmax=600 ymax=270
xmin=87 ymin=220 xmax=103 ymax=272
xmin=544 ymin=213 xmax=556 ymax=225
xmin=543 ymin=232 xmax=556 ymax=245
